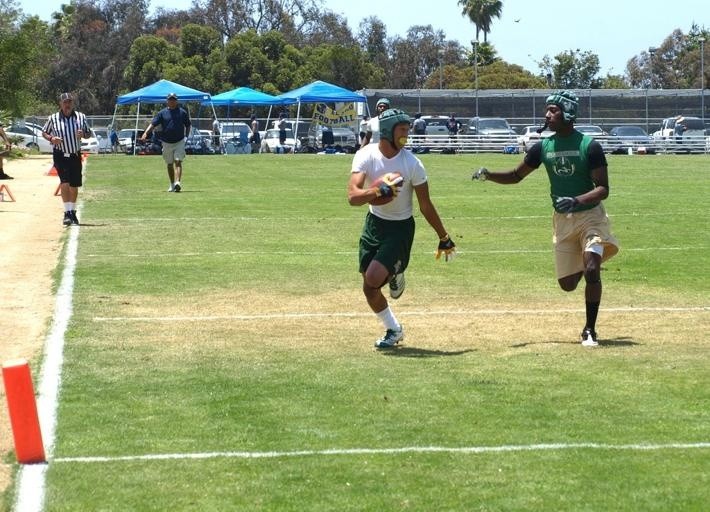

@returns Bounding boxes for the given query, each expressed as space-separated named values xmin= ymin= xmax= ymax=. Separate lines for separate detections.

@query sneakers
xmin=168 ymin=182 xmax=181 ymax=192
xmin=388 ymin=260 xmax=405 ymax=300
xmin=373 ymin=323 xmax=404 ymax=349
xmin=581 ymin=325 xmax=600 ymax=347
xmin=63 ymin=210 xmax=79 ymax=225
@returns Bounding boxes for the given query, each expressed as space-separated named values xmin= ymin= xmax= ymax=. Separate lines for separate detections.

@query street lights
xmin=648 ymin=47 xmax=657 ymax=88
xmin=697 ymin=36 xmax=707 ymax=120
xmin=437 ymin=49 xmax=446 ymax=89
xmin=470 ymin=39 xmax=481 ymax=116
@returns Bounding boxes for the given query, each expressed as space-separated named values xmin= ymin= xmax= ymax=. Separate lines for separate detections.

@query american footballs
xmin=368 ymin=172 xmax=402 ymax=205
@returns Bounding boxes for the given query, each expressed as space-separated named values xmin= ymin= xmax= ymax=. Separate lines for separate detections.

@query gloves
xmin=372 ymin=173 xmax=404 ymax=199
xmin=436 ymin=234 xmax=458 ymax=263
xmin=470 ymin=168 xmax=491 ymax=182
xmin=550 ymin=194 xmax=580 ymax=214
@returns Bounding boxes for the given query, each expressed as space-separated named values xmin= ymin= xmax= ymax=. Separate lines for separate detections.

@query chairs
xmin=233 ymin=131 xmax=248 ymax=154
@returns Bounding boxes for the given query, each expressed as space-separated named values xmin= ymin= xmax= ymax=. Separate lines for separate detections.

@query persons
xmin=278 ymin=113 xmax=287 ymax=145
xmin=360 ymin=98 xmax=390 ymax=148
xmin=675 ymin=117 xmax=687 ymax=145
xmin=359 ymin=115 xmax=367 ymax=140
xmin=446 ymin=112 xmax=462 ymax=141
xmin=348 ymin=109 xmax=456 ymax=348
xmin=212 ymin=115 xmax=220 ymax=145
xmin=42 ymin=93 xmax=91 ymax=224
xmin=249 ymin=114 xmax=260 ymax=154
xmin=142 ymin=93 xmax=191 ymax=192
xmin=412 ymin=112 xmax=426 ymax=136
xmin=0 ymin=124 xmax=13 ymax=180
xmin=472 ymin=90 xmax=609 ymax=346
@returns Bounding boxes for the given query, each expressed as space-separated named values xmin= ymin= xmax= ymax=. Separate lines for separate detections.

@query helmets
xmin=379 ymin=109 xmax=411 ymax=142
xmin=545 ymin=90 xmax=579 ymax=125
xmin=375 ymin=99 xmax=391 ymax=113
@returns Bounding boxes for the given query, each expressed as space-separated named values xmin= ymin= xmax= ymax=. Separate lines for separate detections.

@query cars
xmin=570 ymin=124 xmax=611 ymax=152
xmin=607 ymin=126 xmax=657 ymax=156
xmin=517 ymin=125 xmax=556 ymax=152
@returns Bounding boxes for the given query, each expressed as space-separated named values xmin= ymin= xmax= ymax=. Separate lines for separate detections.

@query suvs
xmin=647 ymin=114 xmax=707 ymax=154
xmin=465 ymin=116 xmax=519 ymax=151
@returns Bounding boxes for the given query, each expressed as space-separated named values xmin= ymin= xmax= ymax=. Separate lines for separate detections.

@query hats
xmin=166 ymin=93 xmax=178 ymax=100
xmin=60 ymin=93 xmax=74 ymax=103
